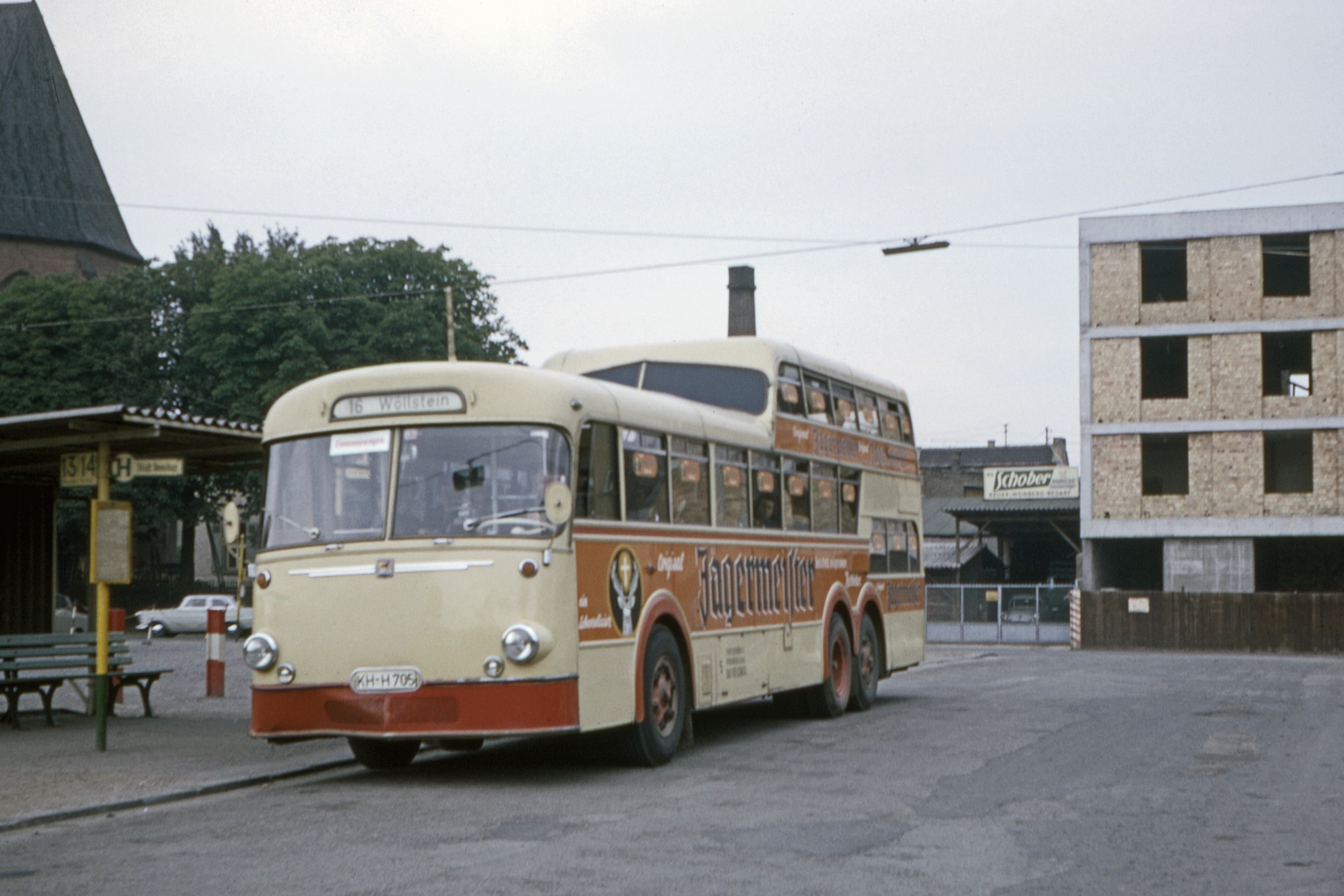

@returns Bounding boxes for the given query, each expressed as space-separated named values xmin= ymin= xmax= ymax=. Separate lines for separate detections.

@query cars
xmin=135 ymin=593 xmax=252 ymax=638
xmin=54 ymin=593 xmax=90 ymax=634
xmin=1002 ymin=592 xmax=1050 ymax=622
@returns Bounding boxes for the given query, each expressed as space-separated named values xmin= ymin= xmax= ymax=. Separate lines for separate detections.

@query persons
xmin=522 ymin=462 xmax=567 ymax=536
xmin=753 ymin=493 xmax=781 ymax=529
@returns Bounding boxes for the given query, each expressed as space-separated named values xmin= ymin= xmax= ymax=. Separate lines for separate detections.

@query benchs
xmin=0 ymin=631 xmax=173 ymax=720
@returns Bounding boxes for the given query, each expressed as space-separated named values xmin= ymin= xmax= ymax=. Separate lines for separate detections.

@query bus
xmin=244 ymin=288 xmax=929 ymax=772
